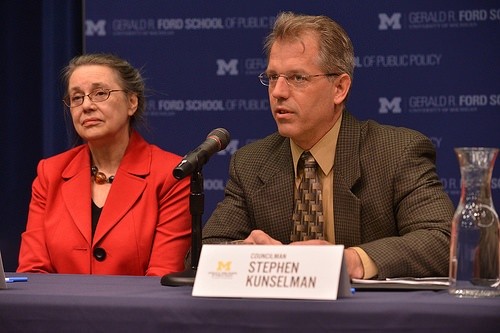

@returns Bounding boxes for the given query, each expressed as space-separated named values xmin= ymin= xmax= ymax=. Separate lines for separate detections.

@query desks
xmin=0 ymin=273 xmax=500 ymax=333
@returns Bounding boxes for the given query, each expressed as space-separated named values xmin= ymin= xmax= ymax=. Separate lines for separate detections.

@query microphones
xmin=173 ymin=127 xmax=231 ymax=179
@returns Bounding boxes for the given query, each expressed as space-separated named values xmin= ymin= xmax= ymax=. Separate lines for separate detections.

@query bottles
xmin=448 ymin=146 xmax=500 ymax=300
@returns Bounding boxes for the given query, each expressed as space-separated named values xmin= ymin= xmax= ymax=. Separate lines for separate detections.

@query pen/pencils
xmin=4 ymin=276 xmax=28 ymax=283
xmin=351 ymin=287 xmax=355 ymax=294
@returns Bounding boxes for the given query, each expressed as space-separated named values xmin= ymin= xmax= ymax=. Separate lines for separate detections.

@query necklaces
xmin=89 ymin=163 xmax=115 ymax=186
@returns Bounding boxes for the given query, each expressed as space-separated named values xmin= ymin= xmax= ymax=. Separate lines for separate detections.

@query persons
xmin=184 ymin=9 xmax=455 ymax=281
xmin=16 ymin=54 xmax=192 ymax=278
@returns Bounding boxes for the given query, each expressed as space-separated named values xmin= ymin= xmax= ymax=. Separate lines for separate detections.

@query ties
xmin=289 ymin=151 xmax=328 ymax=242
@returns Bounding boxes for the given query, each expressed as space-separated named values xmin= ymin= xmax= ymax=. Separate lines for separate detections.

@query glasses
xmin=62 ymin=88 xmax=129 ymax=108
xmin=258 ymin=69 xmax=340 ymax=87
